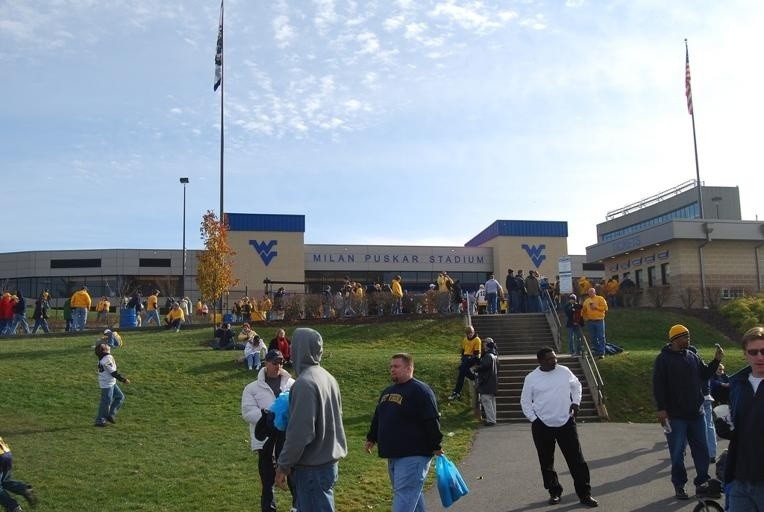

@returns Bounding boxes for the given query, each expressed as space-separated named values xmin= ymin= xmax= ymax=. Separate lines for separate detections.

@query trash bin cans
xmin=120 ymin=307 xmax=136 ymax=328
xmin=223 ymin=313 xmax=232 ymax=323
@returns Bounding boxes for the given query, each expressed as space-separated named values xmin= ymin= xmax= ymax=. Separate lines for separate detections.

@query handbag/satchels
xmin=716 ymin=449 xmax=729 ymax=482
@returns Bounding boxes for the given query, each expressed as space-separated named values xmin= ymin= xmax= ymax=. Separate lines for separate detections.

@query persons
xmin=2 ymin=287 xmax=210 ymax=332
xmin=653 ymin=324 xmax=764 ymax=512
xmin=231 ymin=269 xmax=636 ymax=321
xmin=580 ymin=288 xmax=608 ymax=359
xmin=520 ymin=346 xmax=598 ymax=507
xmin=214 ymin=323 xmax=291 ymax=370
xmin=96 ymin=329 xmax=132 ymax=425
xmin=447 ymin=325 xmax=498 ymax=425
xmin=365 ymin=353 xmax=444 ymax=512
xmin=0 ymin=436 xmax=38 ymax=512
xmin=565 ymin=293 xmax=585 ymax=356
xmin=241 ymin=349 xmax=296 ymax=512
xmin=275 ymin=327 xmax=348 ymax=512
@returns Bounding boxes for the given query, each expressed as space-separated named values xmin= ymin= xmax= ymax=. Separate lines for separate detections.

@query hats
xmin=669 ymin=324 xmax=689 ymax=341
xmin=266 ymin=350 xmax=283 ymax=362
xmin=481 ymin=337 xmax=493 ymax=344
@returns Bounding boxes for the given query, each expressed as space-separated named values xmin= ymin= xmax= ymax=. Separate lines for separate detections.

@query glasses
xmin=746 ymin=349 xmax=764 ymax=356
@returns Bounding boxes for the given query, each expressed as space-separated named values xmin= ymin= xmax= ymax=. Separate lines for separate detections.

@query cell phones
xmin=714 ymin=343 xmax=723 ymax=353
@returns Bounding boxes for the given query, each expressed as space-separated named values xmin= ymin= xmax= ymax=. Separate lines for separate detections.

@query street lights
xmin=179 ymin=178 xmax=188 ymax=298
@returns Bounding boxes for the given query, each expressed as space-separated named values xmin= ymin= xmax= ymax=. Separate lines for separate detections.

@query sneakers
xmin=676 ymin=489 xmax=688 ymax=499
xmin=580 ymin=496 xmax=598 ymax=507
xmin=552 ymin=496 xmax=560 ymax=504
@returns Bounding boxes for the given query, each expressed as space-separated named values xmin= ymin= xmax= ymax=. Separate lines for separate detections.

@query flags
xmin=214 ymin=0 xmax=224 ymax=91
xmin=686 ymin=41 xmax=694 ymax=115
